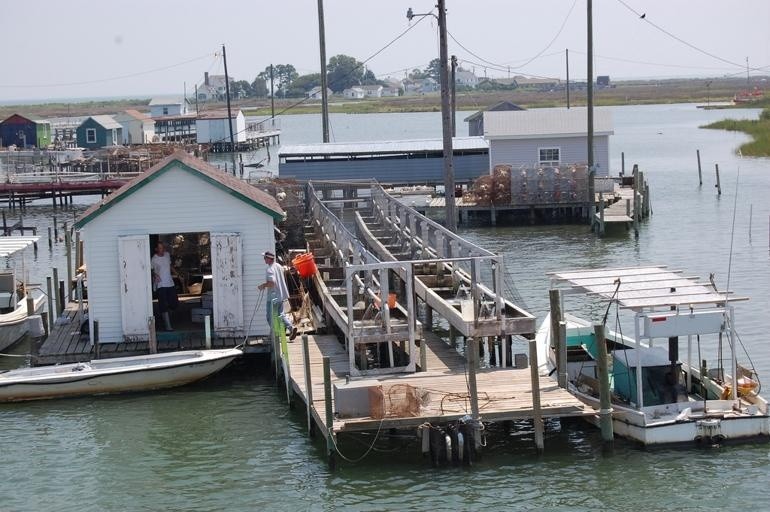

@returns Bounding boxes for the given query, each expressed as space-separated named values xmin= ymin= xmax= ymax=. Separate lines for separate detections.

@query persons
xmin=257 ymin=250 xmax=298 ymax=341
xmin=148 ymin=240 xmax=179 ymax=331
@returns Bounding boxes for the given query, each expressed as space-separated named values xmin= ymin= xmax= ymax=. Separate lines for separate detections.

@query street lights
xmin=407 ymin=8 xmax=438 ymax=23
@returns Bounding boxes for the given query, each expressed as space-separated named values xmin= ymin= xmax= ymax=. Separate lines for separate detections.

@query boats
xmin=0 ymin=236 xmax=46 ymax=352
xmin=0 ymin=349 xmax=243 ymax=402
xmin=536 ymin=266 xmax=769 ymax=451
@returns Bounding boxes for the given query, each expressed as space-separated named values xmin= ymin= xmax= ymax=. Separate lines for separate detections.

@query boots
xmin=162 ymin=311 xmax=181 ymax=331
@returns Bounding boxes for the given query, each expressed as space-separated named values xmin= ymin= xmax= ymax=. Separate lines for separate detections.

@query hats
xmin=262 ymin=250 xmax=275 ymax=259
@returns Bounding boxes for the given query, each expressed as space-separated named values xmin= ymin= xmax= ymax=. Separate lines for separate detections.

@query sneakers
xmin=289 ymin=327 xmax=297 ymax=341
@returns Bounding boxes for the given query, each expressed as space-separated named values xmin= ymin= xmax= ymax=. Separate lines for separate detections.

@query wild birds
xmin=640 ymin=13 xmax=646 ymax=19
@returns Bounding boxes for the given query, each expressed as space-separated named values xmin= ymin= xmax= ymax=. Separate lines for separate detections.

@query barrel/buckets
xmin=292 ymin=252 xmax=318 ymax=277
